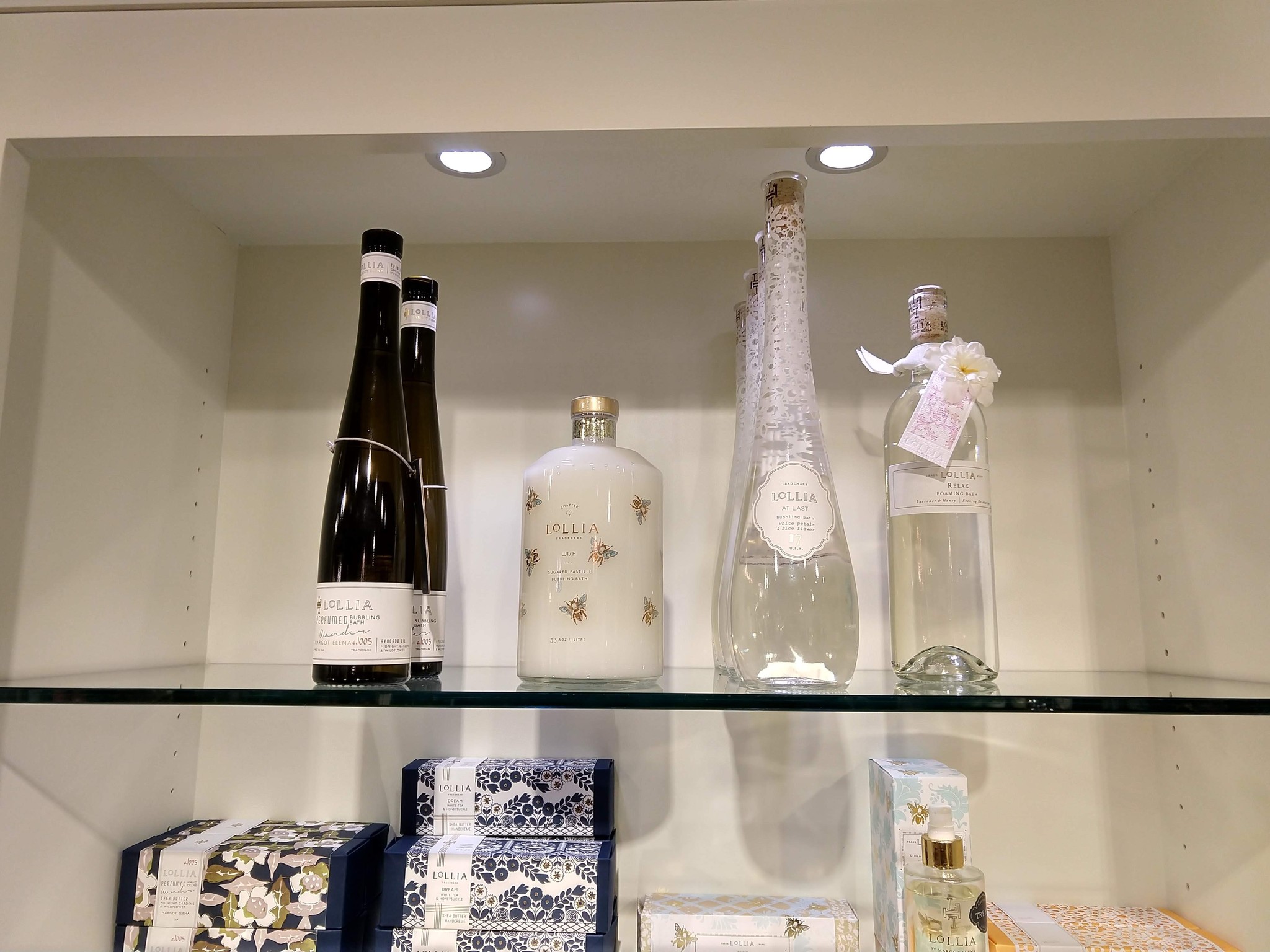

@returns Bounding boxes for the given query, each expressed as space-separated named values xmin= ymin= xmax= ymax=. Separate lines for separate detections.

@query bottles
xmin=311 ymin=231 xmax=448 ymax=687
xmin=515 ymin=395 xmax=665 ymax=684
xmin=904 ymin=803 xmax=990 ymax=952
xmin=884 ymin=284 xmax=999 ymax=684
xmin=717 ymin=172 xmax=860 ymax=690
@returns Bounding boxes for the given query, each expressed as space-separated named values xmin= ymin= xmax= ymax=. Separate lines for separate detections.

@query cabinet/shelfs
xmin=0 ymin=0 xmax=1270 ymax=952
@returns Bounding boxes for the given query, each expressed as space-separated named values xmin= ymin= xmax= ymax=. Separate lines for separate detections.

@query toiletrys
xmin=910 ymin=804 xmax=986 ymax=952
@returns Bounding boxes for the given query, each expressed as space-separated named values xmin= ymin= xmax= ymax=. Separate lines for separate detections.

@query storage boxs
xmin=868 ymin=758 xmax=972 ymax=952
xmin=985 ymin=901 xmax=1241 ymax=952
xmin=636 ymin=893 xmax=861 ymax=952
xmin=114 ymin=759 xmax=617 ymax=952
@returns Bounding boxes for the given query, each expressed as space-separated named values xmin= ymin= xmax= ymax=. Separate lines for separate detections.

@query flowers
xmin=925 ymin=336 xmax=1000 ymax=407
